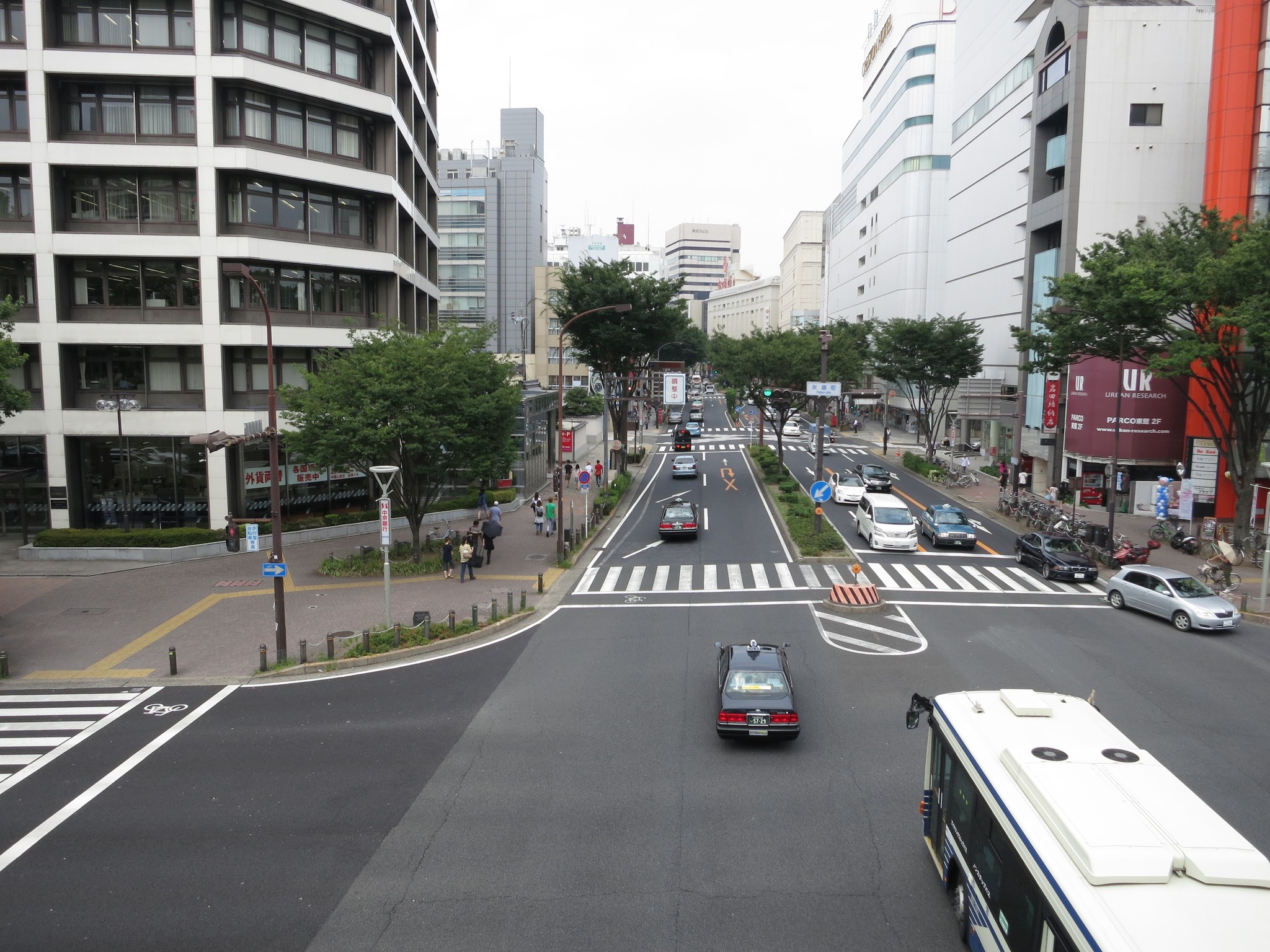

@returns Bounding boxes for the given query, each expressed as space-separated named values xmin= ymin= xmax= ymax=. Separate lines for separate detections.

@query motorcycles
xmin=1110 ymin=539 xmax=1161 ymax=570
xmin=925 ymin=454 xmax=938 ymax=464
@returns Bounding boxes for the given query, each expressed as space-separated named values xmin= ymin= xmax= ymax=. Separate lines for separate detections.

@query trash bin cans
xmin=1086 ymin=523 xmax=1095 ymax=543
xmin=1115 ymin=494 xmax=1122 ymax=507
xmin=1120 ymin=494 xmax=1130 ymax=507
xmin=1094 ymin=525 xmax=1108 ymax=546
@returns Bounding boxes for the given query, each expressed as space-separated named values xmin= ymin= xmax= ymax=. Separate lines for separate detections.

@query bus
xmin=904 ymin=689 xmax=1270 ymax=952
xmin=692 ymin=374 xmax=702 ymax=383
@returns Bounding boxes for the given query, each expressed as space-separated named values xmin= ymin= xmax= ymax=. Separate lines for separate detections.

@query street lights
xmin=555 ymin=303 xmax=634 ymax=559
xmin=220 ymin=261 xmax=289 ymax=666
xmin=947 ymin=411 xmax=958 ymax=471
xmin=95 ymin=393 xmax=141 ymax=534
xmin=368 ymin=465 xmax=401 ymax=629
xmin=657 ymin=341 xmax=684 ymax=408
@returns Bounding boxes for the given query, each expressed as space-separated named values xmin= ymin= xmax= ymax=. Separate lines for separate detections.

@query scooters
xmin=1170 ymin=525 xmax=1196 ymax=556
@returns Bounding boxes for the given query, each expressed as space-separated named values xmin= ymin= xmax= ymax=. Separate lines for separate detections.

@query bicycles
xmin=995 ymin=484 xmax=1140 ymax=565
xmin=840 ymin=416 xmax=866 ymax=432
xmin=1149 ymin=516 xmax=1181 ymax=541
xmin=425 ymin=519 xmax=457 ymax=544
xmin=1193 ymin=526 xmax=1266 ymax=592
xmin=935 ymin=457 xmax=979 ymax=489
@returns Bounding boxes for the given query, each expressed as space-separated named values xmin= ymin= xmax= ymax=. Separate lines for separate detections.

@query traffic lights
xmin=224 ymin=525 xmax=240 ymax=553
xmin=851 ymin=406 xmax=854 ymax=409
xmin=760 ymin=386 xmax=793 ymax=410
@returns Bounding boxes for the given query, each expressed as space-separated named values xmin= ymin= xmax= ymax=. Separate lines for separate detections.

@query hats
xmin=963 ymin=454 xmax=966 ymax=456
xmin=494 ymin=501 xmax=499 ymax=505
xmin=548 ymin=497 xmax=553 ymax=501
xmin=586 ymin=461 xmax=590 ymax=464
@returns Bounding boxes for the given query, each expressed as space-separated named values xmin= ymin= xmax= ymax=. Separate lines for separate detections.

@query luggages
xmin=473 ymin=545 xmax=485 ymax=568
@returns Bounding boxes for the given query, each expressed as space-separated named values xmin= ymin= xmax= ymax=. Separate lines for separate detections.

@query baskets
xmin=1170 ymin=519 xmax=1179 ymax=526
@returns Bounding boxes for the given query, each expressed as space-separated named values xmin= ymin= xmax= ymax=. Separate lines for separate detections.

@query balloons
xmin=1155 ymin=476 xmax=1174 ymax=517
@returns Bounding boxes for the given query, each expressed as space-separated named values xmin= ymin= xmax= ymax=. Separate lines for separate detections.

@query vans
xmin=671 ymin=429 xmax=691 ymax=452
xmin=702 ymin=377 xmax=715 ymax=394
xmin=855 ymin=492 xmax=919 ymax=552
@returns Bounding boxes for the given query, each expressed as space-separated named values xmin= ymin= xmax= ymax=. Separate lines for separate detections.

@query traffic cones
xmin=896 ymin=446 xmax=900 ymax=456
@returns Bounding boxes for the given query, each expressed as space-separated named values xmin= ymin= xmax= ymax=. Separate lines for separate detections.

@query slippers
xmin=448 ymin=575 xmax=453 ymax=578
xmin=446 ymin=577 xmax=448 ymax=579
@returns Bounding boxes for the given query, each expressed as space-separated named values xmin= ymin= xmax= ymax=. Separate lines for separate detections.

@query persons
xmin=530 ymin=492 xmax=557 ymax=538
xmin=998 ymin=459 xmax=1008 ymax=474
xmin=941 ymin=435 xmax=949 ymax=444
xmin=925 ymin=439 xmax=937 ymax=456
xmin=1019 ymin=468 xmax=1028 ymax=495
xmin=442 ymin=487 xmax=502 ymax=584
xmin=565 ymin=459 xmax=603 ymax=490
xmin=1045 ymin=481 xmax=1068 ymax=511
xmin=1208 ymin=554 xmax=1232 ymax=593
xmin=999 ymin=469 xmax=1009 ymax=493
xmin=829 ymin=402 xmax=890 ymax=443
xmin=633 ymin=402 xmax=656 ymax=430
xmin=959 ymin=454 xmax=970 ymax=472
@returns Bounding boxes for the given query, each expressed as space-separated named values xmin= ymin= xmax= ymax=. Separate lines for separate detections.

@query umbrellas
xmin=481 ymin=520 xmax=503 ymax=538
xmin=1218 ymin=540 xmax=1237 ymax=565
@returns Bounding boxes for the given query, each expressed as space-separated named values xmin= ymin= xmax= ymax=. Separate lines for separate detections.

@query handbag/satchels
xmin=463 ymin=553 xmax=472 ymax=559
xmin=530 ymin=498 xmax=536 ymax=508
xmin=1000 ymin=476 xmax=1004 ymax=483
xmin=553 ymin=521 xmax=556 ymax=530
xmin=1198 ymin=566 xmax=1208 ymax=574
xmin=466 ymin=527 xmax=473 ymax=542
xmin=1045 ymin=494 xmax=1052 ymax=500
xmin=477 ymin=499 xmax=483 ymax=506
xmin=578 ymin=468 xmax=581 ymax=475
xmin=536 ymin=508 xmax=543 ymax=517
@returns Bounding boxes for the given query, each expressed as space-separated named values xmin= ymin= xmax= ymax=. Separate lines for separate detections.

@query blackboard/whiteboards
xmin=1200 ymin=516 xmax=1217 ymax=540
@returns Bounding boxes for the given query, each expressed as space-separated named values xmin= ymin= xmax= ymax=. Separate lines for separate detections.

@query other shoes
xmin=551 ymin=533 xmax=554 ymax=535
xmin=487 ymin=561 xmax=490 ymax=565
xmin=477 ymin=518 xmax=482 ymax=520
xmin=470 ymin=577 xmax=476 ymax=580
xmin=536 ymin=531 xmax=539 ymax=535
xmin=461 ymin=580 xmax=464 ymax=583
xmin=540 ymin=532 xmax=542 ymax=535
xmin=1222 ymin=589 xmax=1230 ymax=593
xmin=546 ymin=531 xmax=549 ymax=537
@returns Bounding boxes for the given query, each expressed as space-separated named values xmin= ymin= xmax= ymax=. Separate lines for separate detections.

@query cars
xmin=738 ymin=384 xmax=802 ymax=437
xmin=917 ymin=504 xmax=977 ymax=550
xmin=1 ymin=444 xmax=46 ymax=464
xmin=971 ymin=440 xmax=982 ymax=451
xmin=680 ymin=382 xmax=705 ymax=436
xmin=100 ymin=447 xmax=191 ymax=480
xmin=826 ymin=464 xmax=893 ymax=504
xmin=658 ymin=501 xmax=700 ymax=540
xmin=1013 ymin=532 xmax=1242 ymax=633
xmin=715 ymin=640 xmax=800 ymax=741
xmin=672 ymin=456 xmax=699 ymax=478
xmin=667 ymin=412 xmax=682 ymax=424
